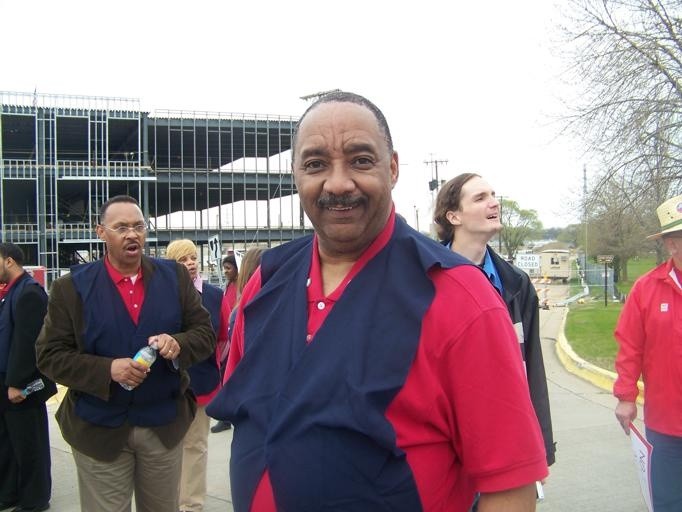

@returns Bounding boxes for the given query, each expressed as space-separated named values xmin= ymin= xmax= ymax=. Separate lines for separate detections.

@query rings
xmin=168 ymin=350 xmax=175 ymax=355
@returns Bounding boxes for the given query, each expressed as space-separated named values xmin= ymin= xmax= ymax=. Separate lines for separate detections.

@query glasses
xmin=102 ymin=219 xmax=149 ymax=233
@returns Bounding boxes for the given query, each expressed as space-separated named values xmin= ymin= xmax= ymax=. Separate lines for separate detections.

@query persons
xmin=163 ymin=237 xmax=231 ymax=512
xmin=0 ymin=239 xmax=61 ymax=512
xmin=610 ymin=226 xmax=680 ymax=509
xmin=210 ymin=252 xmax=238 ymax=436
xmin=202 ymin=87 xmax=553 ymax=512
xmin=33 ymin=193 xmax=220 ymax=512
xmin=430 ymin=170 xmax=560 ymax=500
xmin=227 ymin=245 xmax=270 ymax=347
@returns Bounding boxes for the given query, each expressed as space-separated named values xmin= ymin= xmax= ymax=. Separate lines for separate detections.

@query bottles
xmin=118 ymin=341 xmax=160 ymax=391
xmin=20 ymin=378 xmax=45 ymax=397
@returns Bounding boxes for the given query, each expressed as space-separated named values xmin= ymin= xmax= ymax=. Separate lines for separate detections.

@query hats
xmin=646 ymin=194 xmax=681 ymax=241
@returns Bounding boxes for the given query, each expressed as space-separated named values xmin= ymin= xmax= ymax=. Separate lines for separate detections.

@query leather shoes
xmin=210 ymin=421 xmax=231 ymax=433
